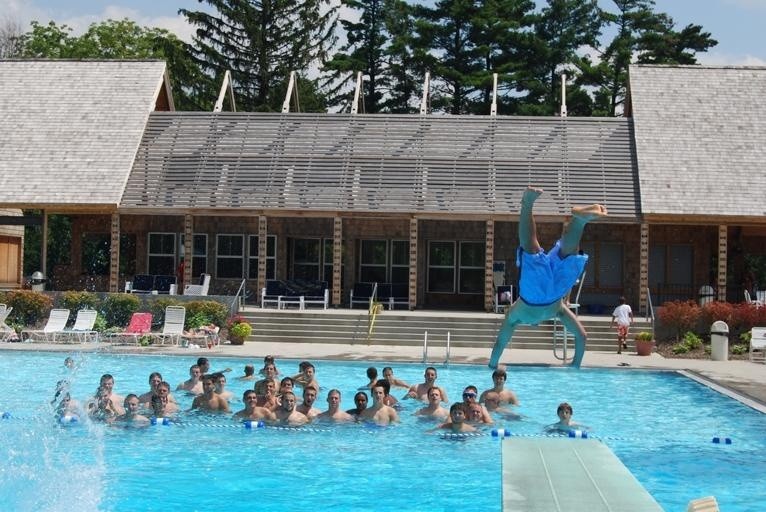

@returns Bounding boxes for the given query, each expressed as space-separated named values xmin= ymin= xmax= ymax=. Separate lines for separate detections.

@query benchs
xmin=125 ymin=273 xmax=178 ymax=295
xmin=262 ymin=279 xmax=330 ymax=310
xmin=349 ymin=282 xmax=410 ymax=310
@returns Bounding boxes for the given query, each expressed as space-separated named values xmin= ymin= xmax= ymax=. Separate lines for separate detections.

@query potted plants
xmin=635 ymin=332 xmax=655 ymax=355
xmin=228 ymin=322 xmax=251 ymax=344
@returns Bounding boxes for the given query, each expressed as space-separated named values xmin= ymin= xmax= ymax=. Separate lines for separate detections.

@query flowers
xmin=227 ymin=313 xmax=246 ymax=330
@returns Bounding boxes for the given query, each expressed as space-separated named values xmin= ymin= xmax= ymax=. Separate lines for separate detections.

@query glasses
xmin=463 ymin=393 xmax=475 ymax=397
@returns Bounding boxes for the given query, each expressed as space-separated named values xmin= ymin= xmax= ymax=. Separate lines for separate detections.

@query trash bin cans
xmin=31 ymin=271 xmax=44 ymax=292
xmin=710 ymin=320 xmax=729 ymax=361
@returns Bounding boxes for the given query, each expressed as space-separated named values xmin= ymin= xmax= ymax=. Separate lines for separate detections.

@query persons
xmin=425 ymin=402 xmax=477 ymax=434
xmin=181 ymin=323 xmax=216 ymax=337
xmin=610 ymin=297 xmax=633 ymax=353
xmin=488 ymin=184 xmax=607 ymax=369
xmin=63 ymin=356 xmax=73 ymax=368
xmin=50 ymin=390 xmax=79 ymax=424
xmin=480 ymin=371 xmax=516 ymax=405
xmin=484 ymin=390 xmax=518 ymax=422
xmin=99 ymin=374 xmax=123 ymax=416
xmin=444 ymin=385 xmax=491 ymax=423
xmin=465 ymin=402 xmax=483 ymax=423
xmin=88 ymin=387 xmax=118 ymax=422
xmin=543 ymin=402 xmax=586 ymax=430
xmin=50 ymin=381 xmax=78 ymax=409
xmin=114 ymin=356 xmax=450 ymax=430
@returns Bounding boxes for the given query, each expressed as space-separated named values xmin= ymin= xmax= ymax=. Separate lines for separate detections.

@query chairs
xmin=0 ymin=304 xmax=15 ymax=342
xmin=21 ymin=309 xmax=69 ymax=343
xmin=744 ymin=289 xmax=766 ymax=310
xmin=152 ymin=306 xmax=186 ymax=346
xmin=183 ymin=272 xmax=211 ymax=296
xmin=748 ymin=327 xmax=766 ymax=364
xmin=181 ymin=325 xmax=220 ymax=348
xmin=53 ymin=309 xmax=99 ymax=343
xmin=108 ymin=312 xmax=151 ymax=345
